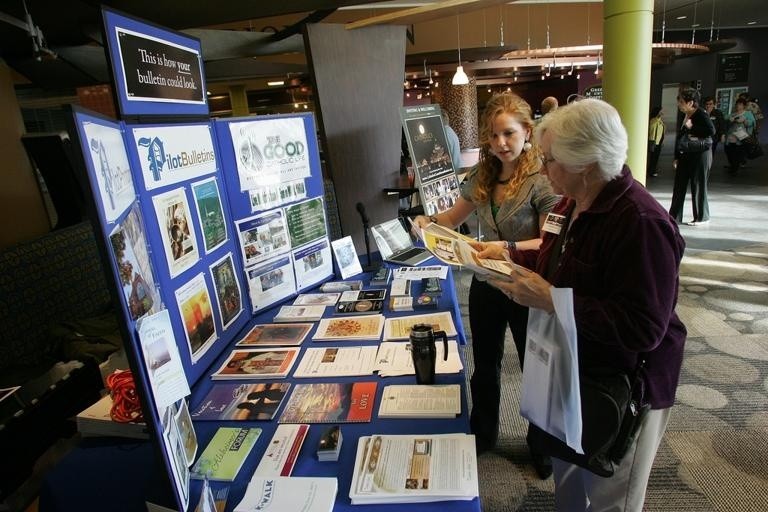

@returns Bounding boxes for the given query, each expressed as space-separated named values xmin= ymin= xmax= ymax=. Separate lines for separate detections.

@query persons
xmin=303 ymin=252 xmax=322 ymax=272
xmin=669 ymin=86 xmax=764 ymax=226
xmin=724 ymin=98 xmax=756 ymax=177
xmin=468 ymin=99 xmax=687 ymax=512
xmin=260 ymin=270 xmax=283 ymax=291
xmin=534 ymin=96 xmax=559 ymax=127
xmin=244 ymin=232 xmax=286 ymax=259
xmin=411 ymin=94 xmax=559 ymax=479
xmin=422 ymin=176 xmax=461 ymax=214
xmin=648 ymin=106 xmax=666 ymax=176
xmin=440 ymin=109 xmax=460 ymax=176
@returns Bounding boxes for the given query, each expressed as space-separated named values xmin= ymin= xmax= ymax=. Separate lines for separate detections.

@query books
xmin=331 ymin=236 xmax=363 ymax=280
xmin=189 ymin=280 xmax=386 ymax=512
xmin=370 ymin=217 xmax=449 ymax=312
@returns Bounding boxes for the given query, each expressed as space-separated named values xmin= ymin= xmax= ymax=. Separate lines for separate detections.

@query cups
xmin=410 ymin=322 xmax=449 ymax=384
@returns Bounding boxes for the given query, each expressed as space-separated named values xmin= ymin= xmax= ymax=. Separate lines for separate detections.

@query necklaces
xmin=494 ymin=175 xmax=512 ymax=186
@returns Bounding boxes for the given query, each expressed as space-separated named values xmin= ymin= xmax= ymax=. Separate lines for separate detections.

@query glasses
xmin=539 ymin=150 xmax=555 ymax=164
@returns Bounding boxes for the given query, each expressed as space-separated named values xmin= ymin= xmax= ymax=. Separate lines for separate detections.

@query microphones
xmin=356 ymin=202 xmax=372 ymax=230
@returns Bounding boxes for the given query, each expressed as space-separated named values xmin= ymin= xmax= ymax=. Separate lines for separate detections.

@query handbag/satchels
xmin=740 ymin=132 xmax=763 ymax=161
xmin=526 ymin=359 xmax=651 ymax=477
xmin=677 ymin=126 xmax=713 ymax=154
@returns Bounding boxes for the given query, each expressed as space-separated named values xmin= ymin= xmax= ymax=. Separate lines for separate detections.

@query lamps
xmin=452 ymin=15 xmax=469 ymax=85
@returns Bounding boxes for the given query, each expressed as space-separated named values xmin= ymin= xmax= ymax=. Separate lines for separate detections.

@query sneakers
xmin=524 ymin=440 xmax=554 ymax=481
xmin=687 ymin=220 xmax=710 ymax=226
xmin=476 ymin=437 xmax=493 ymax=458
